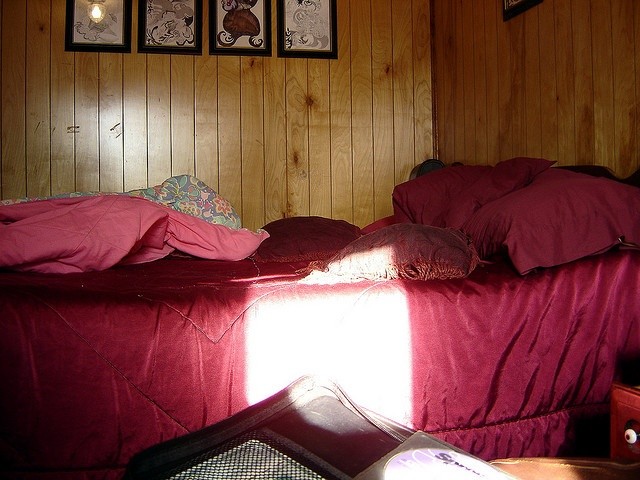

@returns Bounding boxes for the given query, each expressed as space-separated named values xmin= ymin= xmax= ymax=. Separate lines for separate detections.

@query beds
xmin=0 ymin=172 xmax=639 ymax=456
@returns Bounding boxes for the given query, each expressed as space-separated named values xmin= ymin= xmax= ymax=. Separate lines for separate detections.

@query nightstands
xmin=609 ymin=382 xmax=640 ymax=462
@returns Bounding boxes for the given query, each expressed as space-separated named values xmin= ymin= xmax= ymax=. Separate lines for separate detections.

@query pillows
xmin=249 ymin=216 xmax=362 ymax=263
xmin=392 ymin=157 xmax=557 ymax=230
xmin=460 ymin=168 xmax=640 ymax=276
xmin=297 ymin=223 xmax=482 ymax=286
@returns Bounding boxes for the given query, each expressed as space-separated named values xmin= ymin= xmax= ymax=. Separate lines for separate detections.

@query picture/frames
xmin=208 ymin=0 xmax=273 ymax=57
xmin=502 ymin=0 xmax=545 ymax=23
xmin=276 ymin=0 xmax=338 ymax=59
xmin=138 ymin=0 xmax=203 ymax=56
xmin=65 ymin=0 xmax=133 ymax=54
